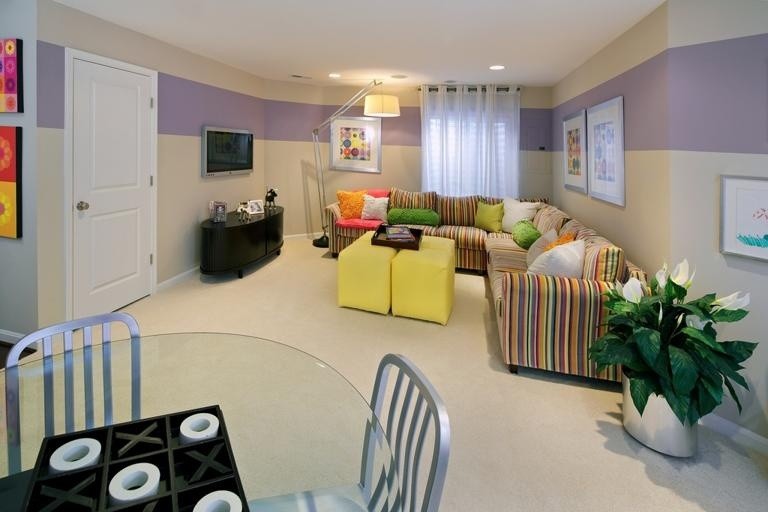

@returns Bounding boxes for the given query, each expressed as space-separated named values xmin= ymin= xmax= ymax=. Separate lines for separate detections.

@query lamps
xmin=312 ymin=80 xmax=401 ymax=248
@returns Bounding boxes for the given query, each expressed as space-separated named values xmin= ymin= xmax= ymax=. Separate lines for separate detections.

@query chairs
xmin=245 ymin=353 xmax=450 ymax=512
xmin=5 ymin=312 xmax=140 ymax=478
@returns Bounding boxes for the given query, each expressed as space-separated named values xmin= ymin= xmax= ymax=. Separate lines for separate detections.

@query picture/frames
xmin=561 ymin=108 xmax=587 ymax=194
xmin=212 ymin=201 xmax=226 ymax=222
xmin=248 ymin=200 xmax=264 ymax=214
xmin=585 ymin=95 xmax=624 ymax=208
xmin=717 ymin=174 xmax=767 ymax=262
xmin=328 ymin=116 xmax=381 ymax=174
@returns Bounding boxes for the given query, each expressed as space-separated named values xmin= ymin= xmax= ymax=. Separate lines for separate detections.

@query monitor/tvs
xmin=201 ymin=126 xmax=254 ymax=179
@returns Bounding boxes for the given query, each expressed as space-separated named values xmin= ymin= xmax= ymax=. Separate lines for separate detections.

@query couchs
xmin=324 ymin=200 xmax=651 ymax=383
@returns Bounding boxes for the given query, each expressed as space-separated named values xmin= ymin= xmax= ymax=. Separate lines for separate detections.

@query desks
xmin=0 ymin=332 xmax=401 ymax=512
xmin=199 ymin=205 xmax=284 ymax=278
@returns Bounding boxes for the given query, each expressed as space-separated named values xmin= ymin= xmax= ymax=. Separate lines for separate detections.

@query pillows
xmin=336 ymin=188 xmax=585 ymax=280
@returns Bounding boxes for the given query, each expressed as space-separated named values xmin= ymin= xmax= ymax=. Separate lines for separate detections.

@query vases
xmin=621 ymin=373 xmax=698 ymax=458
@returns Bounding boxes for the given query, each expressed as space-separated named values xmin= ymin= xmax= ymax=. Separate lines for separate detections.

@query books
xmin=385 ymin=224 xmax=417 ymax=243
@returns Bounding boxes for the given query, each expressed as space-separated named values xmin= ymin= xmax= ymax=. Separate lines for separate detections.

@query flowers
xmin=586 ymin=257 xmax=759 ymax=426
